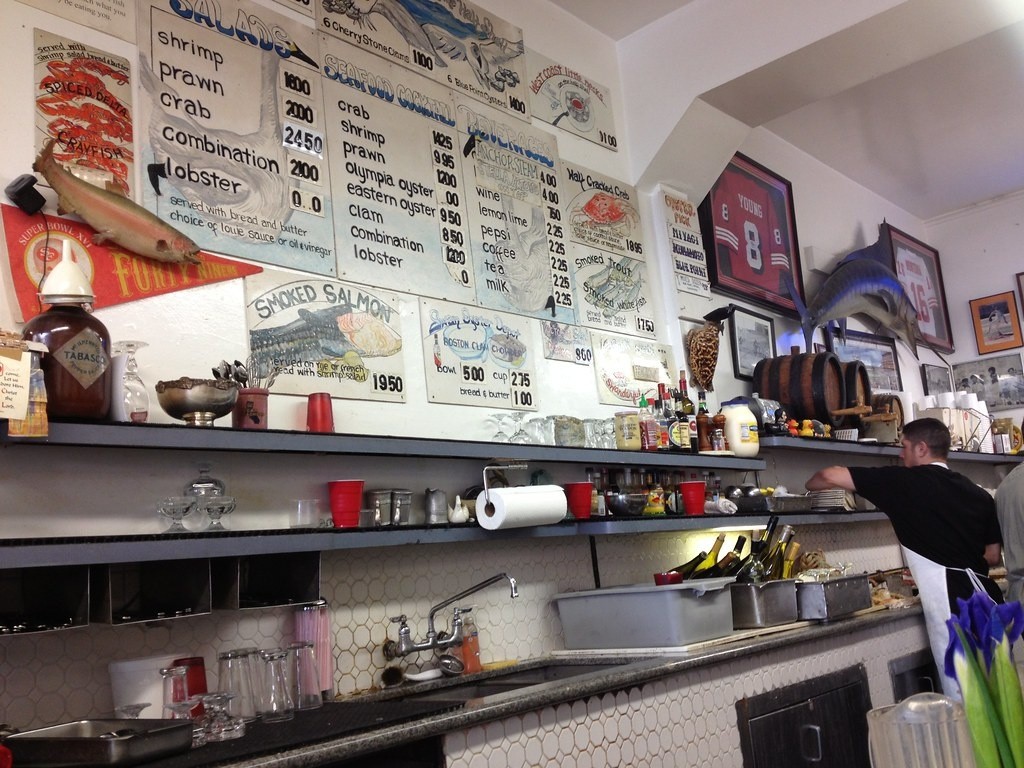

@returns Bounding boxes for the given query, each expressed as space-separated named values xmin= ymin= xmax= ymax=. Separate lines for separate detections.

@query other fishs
xmin=32 ymin=137 xmax=202 ymax=266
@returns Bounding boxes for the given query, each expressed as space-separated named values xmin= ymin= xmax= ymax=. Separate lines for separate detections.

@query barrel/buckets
xmin=873 ymin=393 xmax=905 ymax=430
xmin=841 ymin=361 xmax=873 ymax=429
xmin=753 ymin=346 xmax=847 ymax=427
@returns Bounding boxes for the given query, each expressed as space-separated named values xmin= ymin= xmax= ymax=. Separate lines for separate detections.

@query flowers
xmin=943 ymin=588 xmax=1024 ymax=768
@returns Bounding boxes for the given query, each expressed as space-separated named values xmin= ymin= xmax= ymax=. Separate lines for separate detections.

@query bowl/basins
xmin=604 ymin=494 xmax=649 ymax=515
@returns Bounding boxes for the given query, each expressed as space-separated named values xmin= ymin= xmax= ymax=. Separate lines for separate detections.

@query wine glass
xmin=156 ymin=459 xmax=238 ymax=534
xmin=112 ymin=341 xmax=150 ymax=423
xmin=113 ymin=692 xmax=246 ymax=749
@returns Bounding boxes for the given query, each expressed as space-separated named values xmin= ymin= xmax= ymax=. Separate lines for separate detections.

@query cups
xmin=327 ymin=479 xmax=451 ymax=529
xmin=161 ymin=641 xmax=323 ymax=724
xmin=233 ymin=389 xmax=269 ymax=430
xmin=918 ymin=390 xmax=1012 ymax=453
xmin=653 ymin=572 xmax=682 ymax=585
xmin=564 ymin=482 xmax=593 ymax=518
xmin=306 ymin=393 xmax=336 ymax=433
xmin=678 ymin=481 xmax=706 ymax=516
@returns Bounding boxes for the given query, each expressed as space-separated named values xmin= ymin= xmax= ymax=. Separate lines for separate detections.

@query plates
xmin=806 ymin=488 xmax=860 ymax=512
xmin=736 ymin=496 xmax=812 ymax=514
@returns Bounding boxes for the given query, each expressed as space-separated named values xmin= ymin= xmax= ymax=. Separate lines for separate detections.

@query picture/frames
xmin=922 ymin=363 xmax=952 ymax=395
xmin=969 ymin=290 xmax=1024 ymax=355
xmin=814 ymin=343 xmax=827 ymax=354
xmin=823 ymin=325 xmax=904 ymax=394
xmin=950 ymin=352 xmax=1024 ymax=412
xmin=728 ymin=302 xmax=779 ymax=382
xmin=705 ymin=150 xmax=807 ymax=319
xmin=886 ymin=223 xmax=955 ymax=355
xmin=1016 ymin=271 xmax=1024 ymax=318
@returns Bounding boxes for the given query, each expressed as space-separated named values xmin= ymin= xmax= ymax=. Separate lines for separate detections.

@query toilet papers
xmin=476 ymin=485 xmax=570 ymax=532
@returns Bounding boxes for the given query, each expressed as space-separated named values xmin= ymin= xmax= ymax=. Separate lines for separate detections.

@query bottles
xmin=461 ymin=618 xmax=483 ymax=674
xmin=585 ymin=466 xmax=724 ymax=514
xmin=614 ymin=370 xmax=759 ymax=458
xmin=21 ymin=304 xmax=114 ymax=420
xmin=670 ymin=515 xmax=801 ymax=582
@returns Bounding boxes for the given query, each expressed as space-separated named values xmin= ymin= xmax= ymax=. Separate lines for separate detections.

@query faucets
xmin=383 ymin=571 xmax=519 ymax=661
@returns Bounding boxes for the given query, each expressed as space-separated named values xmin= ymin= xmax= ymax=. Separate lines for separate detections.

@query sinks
xmin=486 ymin=653 xmax=654 ymax=687
xmin=348 ymin=676 xmax=548 ymax=705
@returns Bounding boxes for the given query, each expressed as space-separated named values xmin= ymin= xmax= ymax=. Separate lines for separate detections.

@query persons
xmin=987 ymin=306 xmax=1008 ymax=334
xmin=958 ymin=367 xmax=1024 ymax=406
xmin=775 ymin=409 xmax=788 ymax=430
xmin=805 ymin=417 xmax=1000 ymax=700
xmin=993 ymin=419 xmax=1023 ymax=640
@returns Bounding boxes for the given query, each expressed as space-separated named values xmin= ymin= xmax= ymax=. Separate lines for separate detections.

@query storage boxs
xmin=795 ymin=573 xmax=872 ymax=624
xmin=731 ymin=578 xmax=803 ymax=629
xmin=554 ymin=576 xmax=739 ymax=651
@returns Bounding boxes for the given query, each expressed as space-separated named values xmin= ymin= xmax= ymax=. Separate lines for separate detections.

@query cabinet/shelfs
xmin=757 ymin=434 xmax=1024 ymax=526
xmin=748 ymin=681 xmax=872 ymax=768
xmin=0 ymin=418 xmax=767 ymax=570
